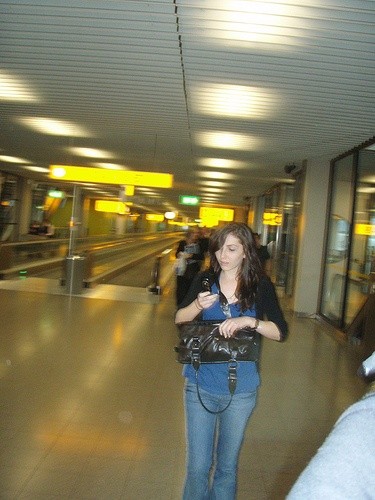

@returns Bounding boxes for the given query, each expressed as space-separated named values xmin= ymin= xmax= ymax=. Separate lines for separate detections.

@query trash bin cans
xmin=61 ymin=253 xmax=87 ymax=297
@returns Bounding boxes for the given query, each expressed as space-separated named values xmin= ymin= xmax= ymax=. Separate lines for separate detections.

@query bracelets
xmin=194 ymin=299 xmax=202 ymax=310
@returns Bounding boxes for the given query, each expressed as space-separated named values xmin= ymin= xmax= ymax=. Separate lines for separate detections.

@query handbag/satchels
xmin=174 ymin=320 xmax=259 ymax=364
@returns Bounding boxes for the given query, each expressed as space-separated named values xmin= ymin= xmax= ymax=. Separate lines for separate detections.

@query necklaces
xmin=224 ymin=291 xmax=237 ymax=304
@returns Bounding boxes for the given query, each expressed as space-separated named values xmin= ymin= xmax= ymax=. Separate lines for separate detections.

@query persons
xmin=174 ymin=222 xmax=288 ymax=500
xmin=285 ymin=352 xmax=375 ymax=500
xmin=175 ymin=225 xmax=270 ymax=313
xmin=27 ymin=217 xmax=55 ymax=259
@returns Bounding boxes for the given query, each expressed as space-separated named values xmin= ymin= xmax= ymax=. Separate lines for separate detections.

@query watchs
xmin=250 ymin=317 xmax=260 ymax=330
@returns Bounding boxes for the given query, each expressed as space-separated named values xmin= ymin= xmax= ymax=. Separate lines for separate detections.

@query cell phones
xmin=202 ymin=277 xmax=211 ymax=293
